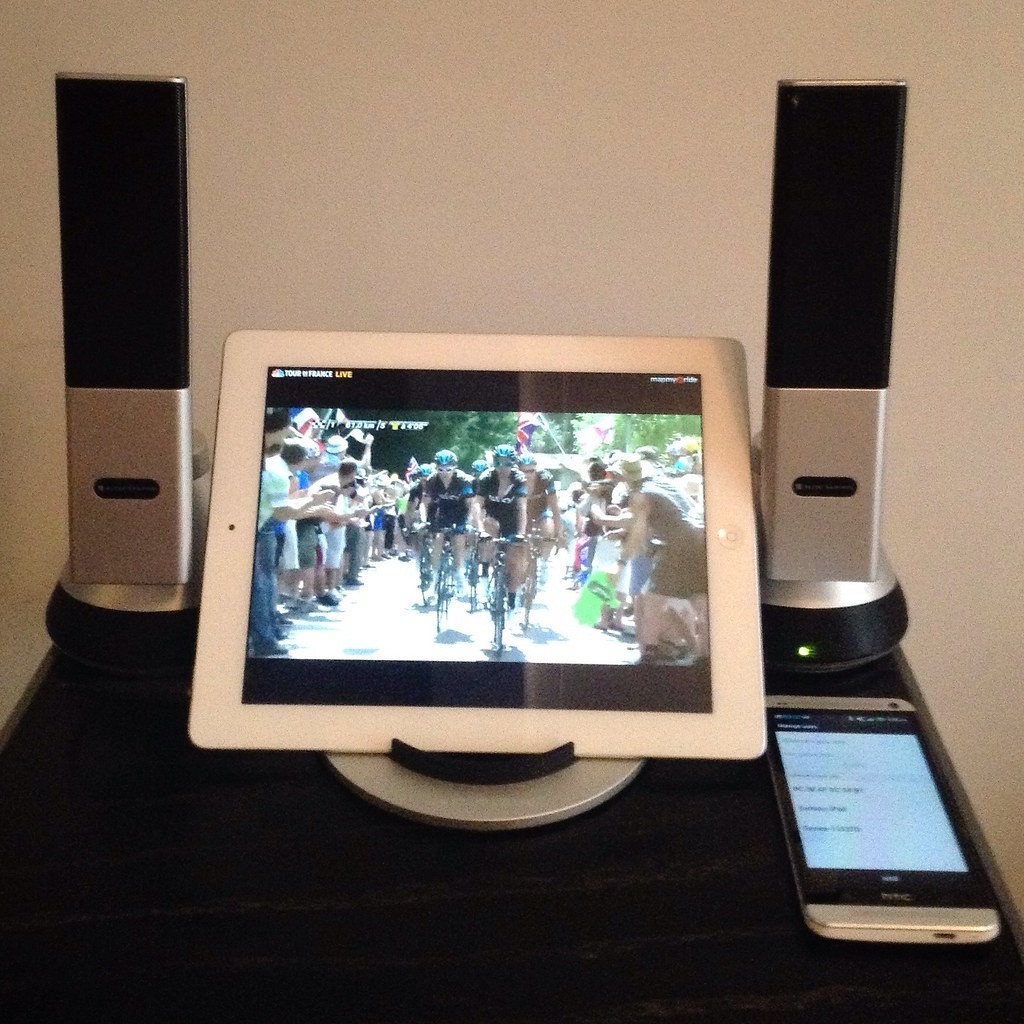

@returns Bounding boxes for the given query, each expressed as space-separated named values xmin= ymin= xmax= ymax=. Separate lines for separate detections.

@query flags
xmin=515 ymin=412 xmax=544 ymax=452
xmin=351 ymin=426 xmax=374 ymax=445
xmin=575 ymin=416 xmax=615 ymax=449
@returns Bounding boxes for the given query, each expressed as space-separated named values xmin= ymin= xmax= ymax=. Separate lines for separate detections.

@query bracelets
xmin=617 ymin=558 xmax=627 ymax=565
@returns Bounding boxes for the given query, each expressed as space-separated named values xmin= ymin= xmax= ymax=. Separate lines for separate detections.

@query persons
xmin=248 ymin=408 xmax=425 ymax=656
xmin=570 ymin=436 xmax=710 ymax=658
xmin=404 ymin=443 xmax=563 ymax=608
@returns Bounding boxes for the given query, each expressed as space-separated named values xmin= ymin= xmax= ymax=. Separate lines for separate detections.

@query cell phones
xmin=765 ymin=695 xmax=1001 ymax=944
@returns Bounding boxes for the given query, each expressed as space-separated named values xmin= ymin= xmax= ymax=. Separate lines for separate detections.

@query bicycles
xmin=415 ymin=526 xmax=558 ymax=652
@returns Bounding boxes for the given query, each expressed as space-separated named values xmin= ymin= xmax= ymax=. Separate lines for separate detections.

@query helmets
xmin=518 ymin=452 xmax=537 ymax=466
xmin=493 ymin=445 xmax=516 ymax=461
xmin=418 ymin=464 xmax=435 ymax=476
xmin=471 ymin=459 xmax=488 ymax=473
xmin=436 ymin=450 xmax=457 ymax=465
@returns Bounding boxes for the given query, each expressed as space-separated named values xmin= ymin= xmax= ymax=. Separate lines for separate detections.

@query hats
xmin=327 ymin=435 xmax=350 ymax=453
xmin=604 ymin=453 xmax=654 ymax=480
xmin=305 ymin=440 xmax=322 ymax=459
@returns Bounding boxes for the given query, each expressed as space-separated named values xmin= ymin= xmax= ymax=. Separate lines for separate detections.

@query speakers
xmin=752 ymin=76 xmax=914 ymax=670
xmin=46 ymin=73 xmax=208 ymax=674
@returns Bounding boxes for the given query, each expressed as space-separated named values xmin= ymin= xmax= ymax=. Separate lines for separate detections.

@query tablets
xmin=188 ymin=329 xmax=769 ymax=761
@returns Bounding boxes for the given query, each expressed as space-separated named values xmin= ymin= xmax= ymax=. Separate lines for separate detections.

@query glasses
xmin=522 ymin=469 xmax=535 ymax=474
xmin=438 ymin=468 xmax=453 ymax=474
xmin=496 ymin=462 xmax=513 ymax=468
